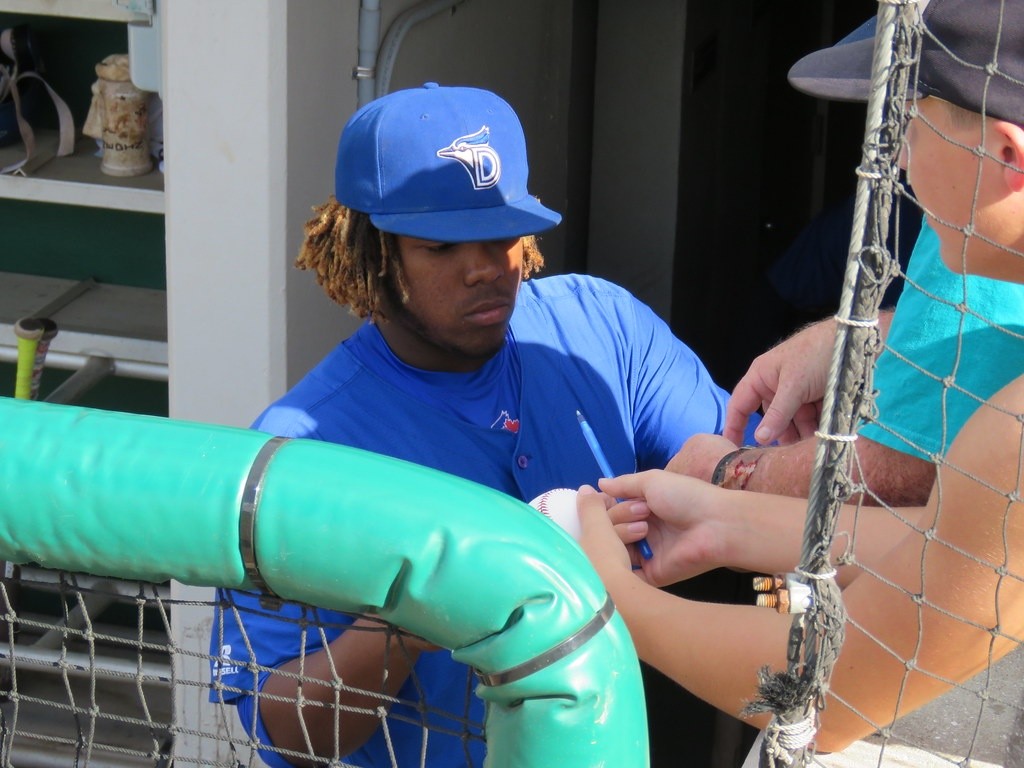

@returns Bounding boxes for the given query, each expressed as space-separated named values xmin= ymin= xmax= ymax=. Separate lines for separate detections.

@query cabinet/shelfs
xmin=1 ymin=1 xmax=171 ymax=608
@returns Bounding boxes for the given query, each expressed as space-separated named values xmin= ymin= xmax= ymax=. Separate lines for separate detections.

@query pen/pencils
xmin=574 ymin=408 xmax=655 ymax=558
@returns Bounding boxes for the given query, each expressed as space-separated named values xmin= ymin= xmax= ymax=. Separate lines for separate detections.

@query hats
xmin=336 ymin=82 xmax=564 ymax=242
xmin=787 ymin=0 xmax=1024 ymax=133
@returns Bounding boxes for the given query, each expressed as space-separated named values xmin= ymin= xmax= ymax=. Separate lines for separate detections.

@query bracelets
xmin=711 ymin=446 xmax=764 ymax=491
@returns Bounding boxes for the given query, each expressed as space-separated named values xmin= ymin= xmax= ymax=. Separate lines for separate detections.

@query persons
xmin=210 ymin=82 xmax=785 ymax=768
xmin=664 ymin=209 xmax=1024 ymax=509
xmin=579 ymin=0 xmax=1024 ymax=752
